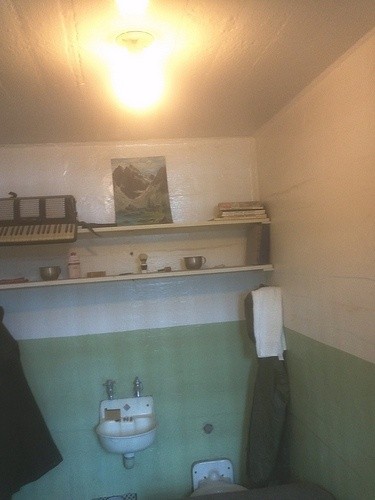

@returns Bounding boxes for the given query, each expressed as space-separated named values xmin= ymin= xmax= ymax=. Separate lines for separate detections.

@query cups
xmin=184 ymin=256 xmax=206 ymax=270
xmin=40 ymin=266 xmax=61 ymax=280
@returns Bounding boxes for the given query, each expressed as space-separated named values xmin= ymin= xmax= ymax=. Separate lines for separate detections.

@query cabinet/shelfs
xmin=0 ymin=218 xmax=272 ymax=289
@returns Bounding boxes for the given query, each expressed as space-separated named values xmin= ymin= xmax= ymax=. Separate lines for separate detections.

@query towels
xmin=251 ymin=286 xmax=287 ymax=360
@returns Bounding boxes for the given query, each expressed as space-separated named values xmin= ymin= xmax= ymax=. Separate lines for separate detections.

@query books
xmin=213 ymin=201 xmax=267 ymax=220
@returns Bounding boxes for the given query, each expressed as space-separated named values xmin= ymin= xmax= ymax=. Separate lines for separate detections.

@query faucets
xmin=133 ymin=377 xmax=142 ymax=397
xmin=102 ymin=379 xmax=116 ymax=399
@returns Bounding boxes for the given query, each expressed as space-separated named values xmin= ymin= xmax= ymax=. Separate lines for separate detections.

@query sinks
xmin=94 ymin=417 xmax=159 ymax=455
